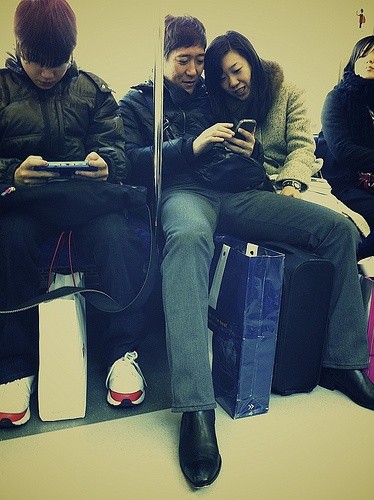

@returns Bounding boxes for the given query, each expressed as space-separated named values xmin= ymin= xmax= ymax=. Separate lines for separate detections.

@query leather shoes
xmin=177 ymin=409 xmax=222 ymax=490
xmin=320 ymin=366 xmax=374 ymax=411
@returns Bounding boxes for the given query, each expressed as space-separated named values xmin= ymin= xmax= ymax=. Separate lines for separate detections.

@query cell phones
xmin=225 ymin=119 xmax=258 ymax=151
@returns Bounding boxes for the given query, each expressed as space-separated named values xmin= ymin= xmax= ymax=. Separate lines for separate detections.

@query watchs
xmin=282 ymin=180 xmax=302 ymax=193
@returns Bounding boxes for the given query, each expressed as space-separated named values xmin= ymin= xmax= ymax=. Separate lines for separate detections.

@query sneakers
xmin=104 ymin=352 xmax=147 ymax=407
xmin=0 ymin=375 xmax=35 ymax=428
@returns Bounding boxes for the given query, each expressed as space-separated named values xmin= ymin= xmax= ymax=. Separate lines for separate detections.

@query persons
xmin=117 ymin=14 xmax=374 ymax=489
xmin=315 ymin=35 xmax=374 ymax=260
xmin=204 ymin=29 xmax=371 ymax=237
xmin=0 ymin=0 xmax=156 ymax=426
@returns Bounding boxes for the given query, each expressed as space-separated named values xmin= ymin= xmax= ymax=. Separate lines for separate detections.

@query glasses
xmin=17 ymin=47 xmax=74 ymax=71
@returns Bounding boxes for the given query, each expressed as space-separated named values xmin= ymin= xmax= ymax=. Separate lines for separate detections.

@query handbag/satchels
xmin=36 ymin=229 xmax=90 ymax=421
xmin=7 ymin=178 xmax=149 ymax=225
xmin=207 ymin=233 xmax=285 ymax=420
xmin=190 ymin=149 xmax=266 ymax=191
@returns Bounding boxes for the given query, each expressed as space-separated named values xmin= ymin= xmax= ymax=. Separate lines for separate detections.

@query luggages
xmin=261 ymin=234 xmax=336 ymax=399
